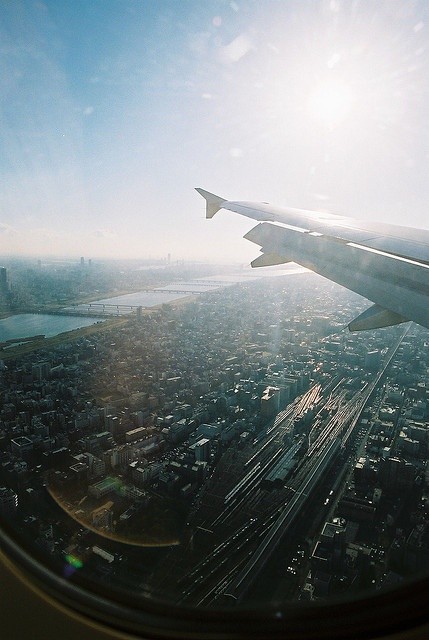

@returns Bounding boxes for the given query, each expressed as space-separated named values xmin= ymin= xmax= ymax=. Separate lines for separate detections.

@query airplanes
xmin=194 ymin=188 xmax=429 ymax=332
